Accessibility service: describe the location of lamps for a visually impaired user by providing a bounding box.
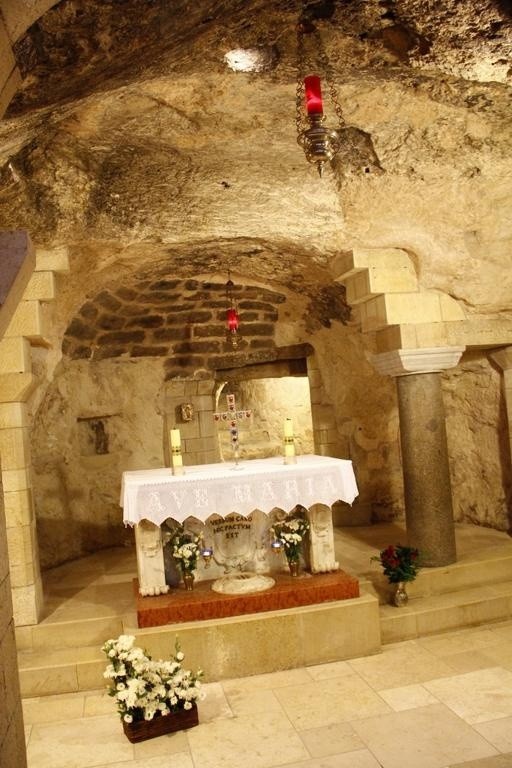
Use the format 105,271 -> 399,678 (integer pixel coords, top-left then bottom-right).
282,416 -> 298,464
220,268 -> 244,353
167,424 -> 185,476
290,0 -> 351,172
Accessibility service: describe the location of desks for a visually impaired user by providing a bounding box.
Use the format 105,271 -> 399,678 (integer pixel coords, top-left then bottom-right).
117,453 -> 359,599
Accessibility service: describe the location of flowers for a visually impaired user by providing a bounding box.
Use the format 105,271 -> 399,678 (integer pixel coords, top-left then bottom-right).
267,513 -> 311,560
369,543 -> 423,583
102,631 -> 208,724
168,530 -> 207,573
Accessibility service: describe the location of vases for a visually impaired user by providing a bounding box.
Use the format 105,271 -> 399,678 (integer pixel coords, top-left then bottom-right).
392,580 -> 408,607
121,701 -> 200,745
182,568 -> 194,590
285,554 -> 301,576
